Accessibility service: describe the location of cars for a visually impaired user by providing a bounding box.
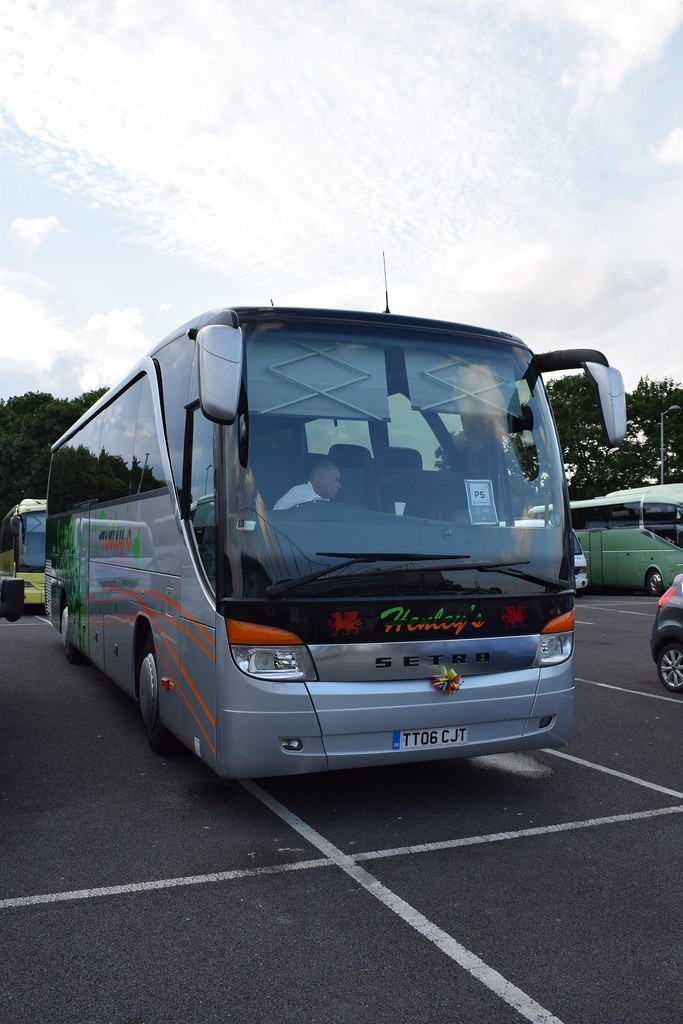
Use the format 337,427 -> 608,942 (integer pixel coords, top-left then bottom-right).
650,573 -> 683,692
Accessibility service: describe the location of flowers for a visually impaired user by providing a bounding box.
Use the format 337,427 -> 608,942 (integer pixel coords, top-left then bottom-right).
431,665 -> 464,696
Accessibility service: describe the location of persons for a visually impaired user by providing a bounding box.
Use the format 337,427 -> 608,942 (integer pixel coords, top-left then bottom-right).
272,463 -> 341,510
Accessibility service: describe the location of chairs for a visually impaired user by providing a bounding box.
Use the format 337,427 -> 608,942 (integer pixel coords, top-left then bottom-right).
328,443 -> 423,472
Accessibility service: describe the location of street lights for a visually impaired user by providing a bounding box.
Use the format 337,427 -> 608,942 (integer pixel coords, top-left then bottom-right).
660,405 -> 680,485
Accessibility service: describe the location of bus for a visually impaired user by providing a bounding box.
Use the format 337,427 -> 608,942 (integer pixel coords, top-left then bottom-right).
527,483 -> 683,597
43,304 -> 627,779
0,498 -> 47,606
